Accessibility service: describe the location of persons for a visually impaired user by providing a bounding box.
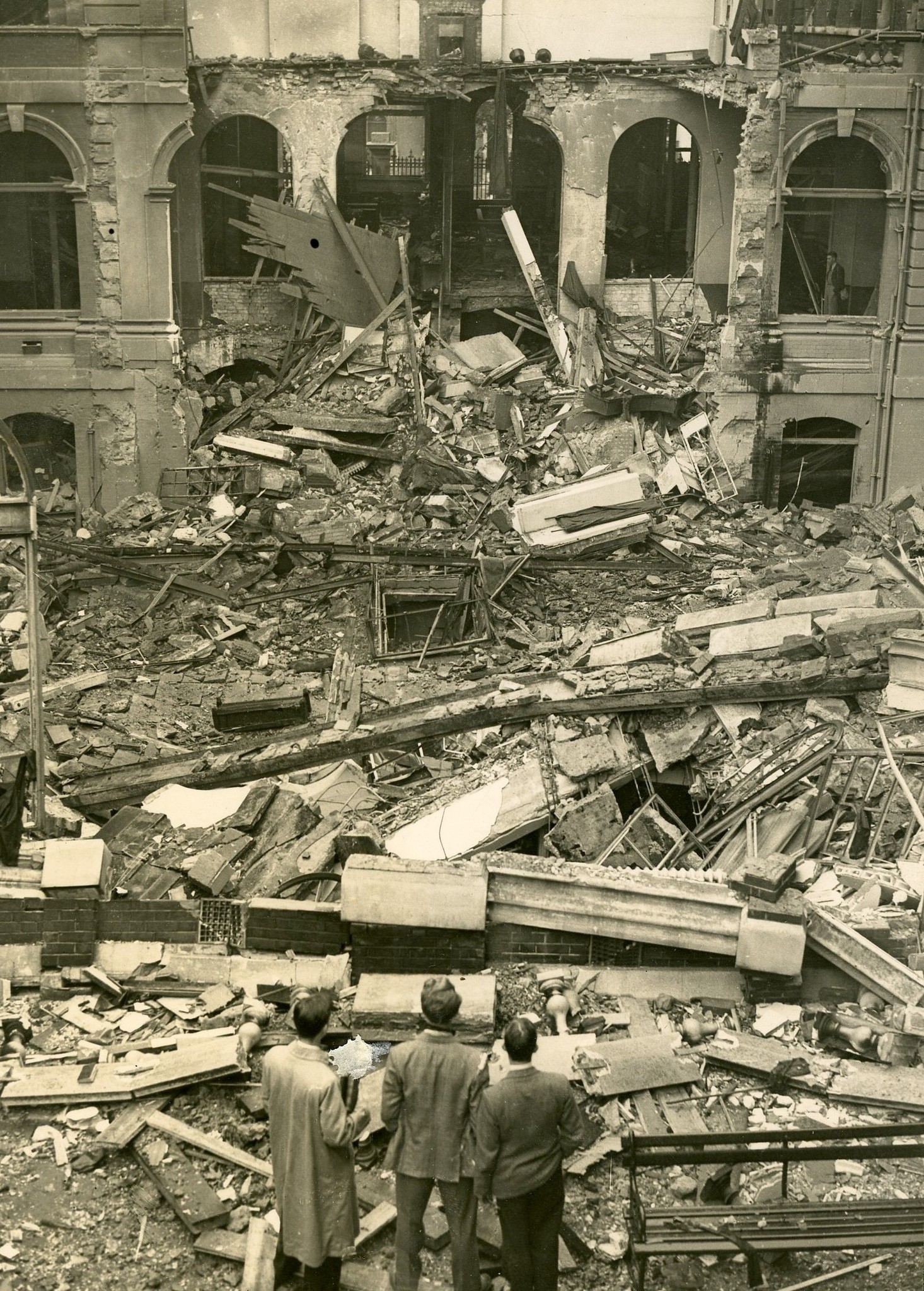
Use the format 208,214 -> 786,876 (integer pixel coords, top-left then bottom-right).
380,978 -> 489,1291
475,1017 -> 584,1291
261,993 -> 372,1291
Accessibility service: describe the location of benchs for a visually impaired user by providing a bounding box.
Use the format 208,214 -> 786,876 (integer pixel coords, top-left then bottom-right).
620,1124 -> 924,1291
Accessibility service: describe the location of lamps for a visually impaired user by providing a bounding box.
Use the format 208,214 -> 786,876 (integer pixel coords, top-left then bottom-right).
22,344 -> 43,353
358,44 -> 375,58
535,49 -> 551,63
508,49 -> 525,63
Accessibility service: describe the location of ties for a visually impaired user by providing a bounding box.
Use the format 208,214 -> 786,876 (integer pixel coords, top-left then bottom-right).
826,265 -> 832,279
